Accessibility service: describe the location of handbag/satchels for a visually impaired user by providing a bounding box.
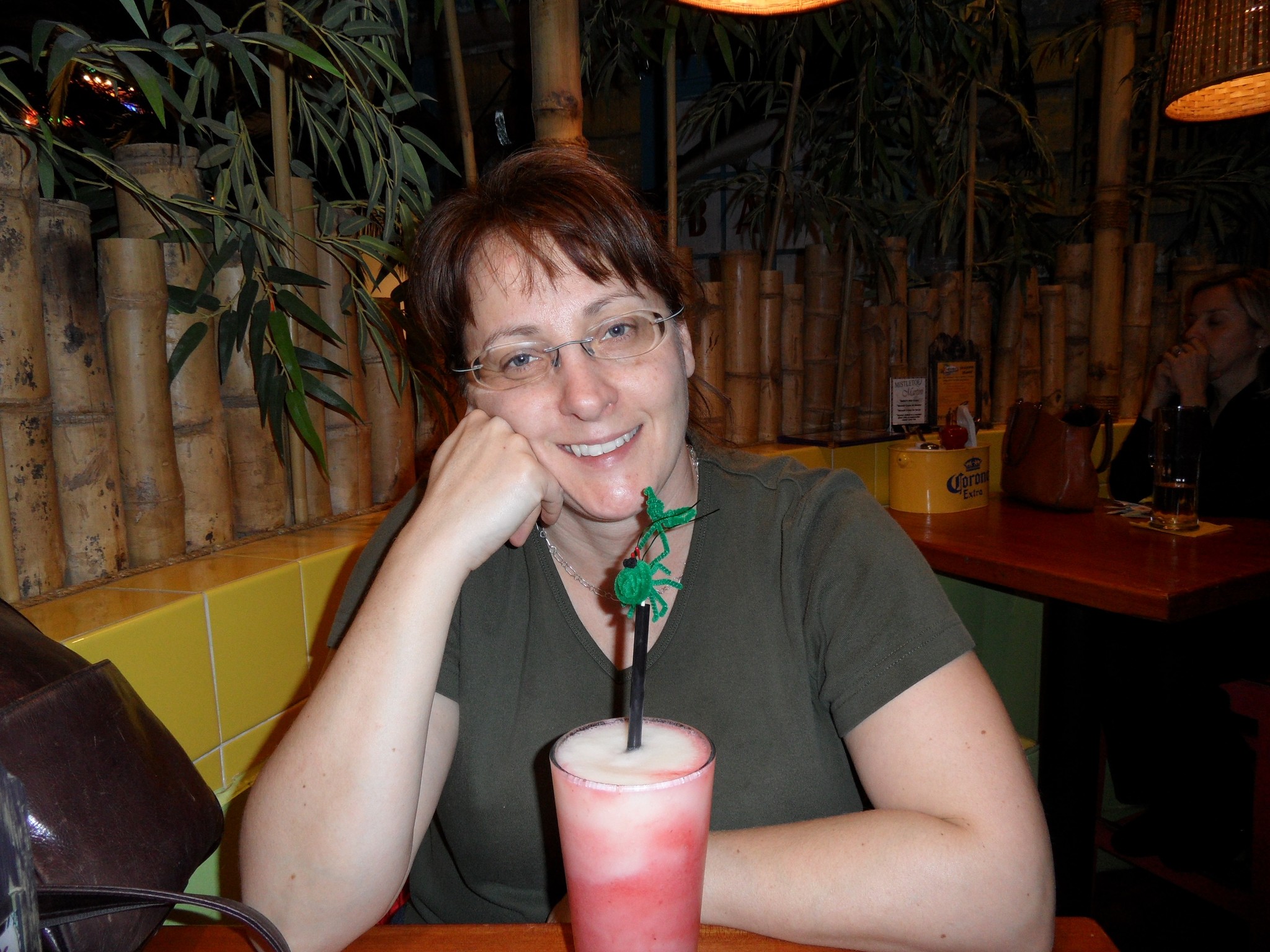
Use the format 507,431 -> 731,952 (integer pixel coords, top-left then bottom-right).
1000,396 -> 1113,510
0,597 -> 292,950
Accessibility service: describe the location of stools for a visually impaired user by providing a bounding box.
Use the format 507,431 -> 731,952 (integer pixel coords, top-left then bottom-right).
1094,668 -> 1270,912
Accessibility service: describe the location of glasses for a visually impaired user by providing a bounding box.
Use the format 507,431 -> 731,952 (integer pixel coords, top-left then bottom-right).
444,303 -> 688,394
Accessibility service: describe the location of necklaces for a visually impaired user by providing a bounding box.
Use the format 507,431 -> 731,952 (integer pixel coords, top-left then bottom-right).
538,444 -> 700,608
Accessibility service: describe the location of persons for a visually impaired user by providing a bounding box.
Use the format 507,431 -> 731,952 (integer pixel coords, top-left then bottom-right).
242,139 -> 1056,952
1110,276 -> 1270,889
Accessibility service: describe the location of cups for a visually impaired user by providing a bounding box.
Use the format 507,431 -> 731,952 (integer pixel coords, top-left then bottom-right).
1150,405 -> 1207,531
549,716 -> 716,952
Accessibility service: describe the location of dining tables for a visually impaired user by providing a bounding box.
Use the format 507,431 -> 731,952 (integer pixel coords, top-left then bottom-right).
137,917 -> 1115,952
863,488 -> 1270,919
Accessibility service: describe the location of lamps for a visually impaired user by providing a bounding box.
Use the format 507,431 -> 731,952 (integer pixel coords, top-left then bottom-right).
1160,0 -> 1270,123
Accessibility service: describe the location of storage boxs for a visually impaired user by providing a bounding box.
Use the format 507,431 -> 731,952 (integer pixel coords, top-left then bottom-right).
888,439 -> 992,514
